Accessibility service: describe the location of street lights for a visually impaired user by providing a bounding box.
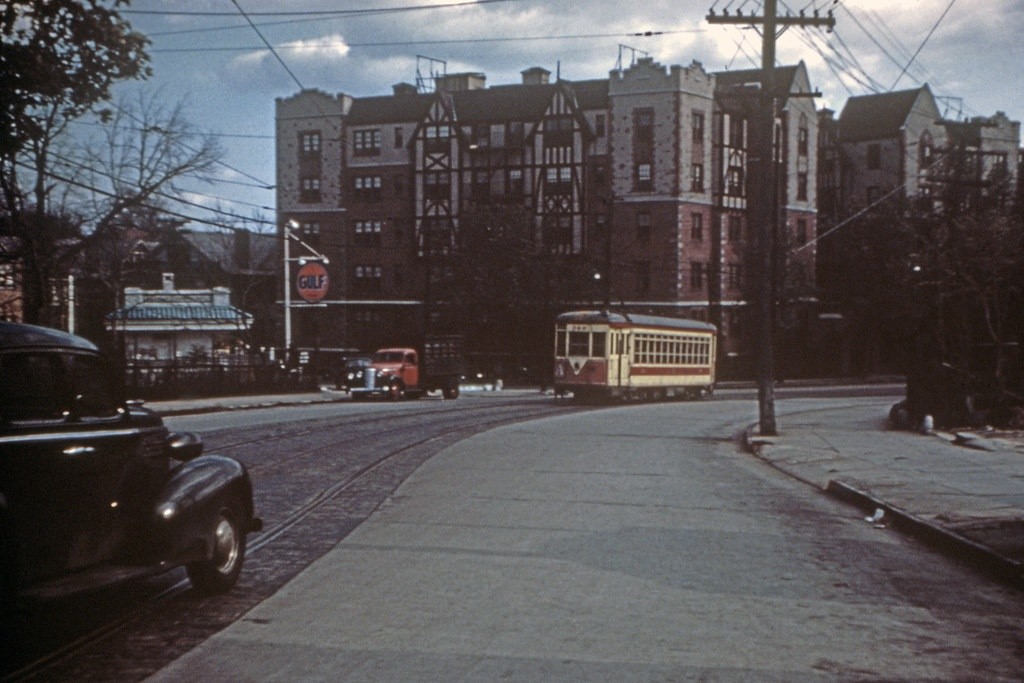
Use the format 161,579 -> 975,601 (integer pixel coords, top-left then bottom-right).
283,219 -> 329,366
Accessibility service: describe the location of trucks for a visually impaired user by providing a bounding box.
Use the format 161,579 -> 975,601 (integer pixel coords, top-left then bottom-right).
364,336 -> 466,399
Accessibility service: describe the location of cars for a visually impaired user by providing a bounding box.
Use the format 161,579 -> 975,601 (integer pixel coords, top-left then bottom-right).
0,322 -> 263,617
337,357 -> 369,390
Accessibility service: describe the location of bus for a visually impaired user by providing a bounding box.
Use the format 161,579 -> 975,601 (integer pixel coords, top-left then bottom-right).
553,262 -> 717,401
